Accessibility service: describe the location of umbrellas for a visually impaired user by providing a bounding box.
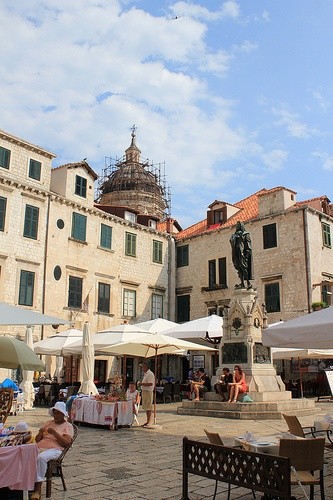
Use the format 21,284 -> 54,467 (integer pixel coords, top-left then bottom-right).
0,302 -> 99,409
99,332 -> 220,425
60,314 -> 223,388
262,305 -> 333,398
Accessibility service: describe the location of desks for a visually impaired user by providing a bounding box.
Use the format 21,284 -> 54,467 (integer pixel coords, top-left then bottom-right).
0,442 -> 40,500
65,395 -> 134,428
155,386 -> 164,397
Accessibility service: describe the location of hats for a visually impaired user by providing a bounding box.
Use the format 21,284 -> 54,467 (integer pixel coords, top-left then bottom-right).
12,422 -> 28,433
48,402 -> 69,418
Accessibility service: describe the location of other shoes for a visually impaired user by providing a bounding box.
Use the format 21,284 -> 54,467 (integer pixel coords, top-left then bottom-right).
141,423 -> 149,428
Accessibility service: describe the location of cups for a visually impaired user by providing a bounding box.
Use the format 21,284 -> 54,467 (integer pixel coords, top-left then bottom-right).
44,427 -> 49,435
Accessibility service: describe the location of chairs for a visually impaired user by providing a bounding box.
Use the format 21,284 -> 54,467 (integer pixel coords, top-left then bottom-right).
44,423 -> 78,496
205,431 -> 257,500
279,437 -> 325,500
158,381 -> 183,403
36,384 -> 57,407
282,414 -> 333,448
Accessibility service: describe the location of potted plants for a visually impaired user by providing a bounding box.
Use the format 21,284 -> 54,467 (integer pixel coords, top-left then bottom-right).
312,302 -> 326,311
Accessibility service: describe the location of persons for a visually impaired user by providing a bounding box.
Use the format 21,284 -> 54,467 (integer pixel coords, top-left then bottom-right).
138,363 -> 155,425
30,402 -> 74,500
230,221 -> 254,289
188,365 -> 247,403
125,384 -> 140,419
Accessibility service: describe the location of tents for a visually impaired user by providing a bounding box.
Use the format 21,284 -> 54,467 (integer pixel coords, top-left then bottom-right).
270,347 -> 333,398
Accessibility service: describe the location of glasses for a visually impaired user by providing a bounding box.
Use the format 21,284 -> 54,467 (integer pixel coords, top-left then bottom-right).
234,369 -> 238,370
